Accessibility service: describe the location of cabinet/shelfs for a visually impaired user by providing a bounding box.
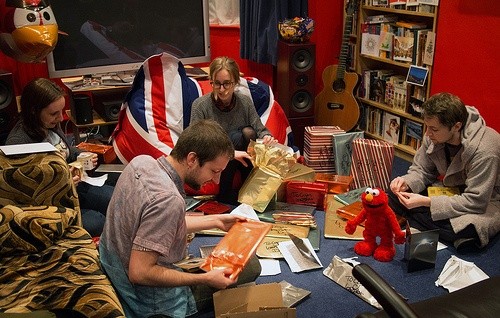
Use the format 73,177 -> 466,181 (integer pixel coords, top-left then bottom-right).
61,64 -> 209,140
353,0 -> 440,163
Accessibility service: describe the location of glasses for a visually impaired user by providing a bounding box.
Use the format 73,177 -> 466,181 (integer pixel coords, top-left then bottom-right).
211,81 -> 237,89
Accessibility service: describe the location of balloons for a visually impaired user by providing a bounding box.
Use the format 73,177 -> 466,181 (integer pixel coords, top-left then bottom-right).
0,0 -> 68,64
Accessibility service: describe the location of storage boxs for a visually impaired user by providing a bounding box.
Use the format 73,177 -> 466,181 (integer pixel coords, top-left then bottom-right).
349,139 -> 394,193
286,181 -> 329,206
302,126 -> 346,174
75,142 -> 116,164
314,173 -> 352,194
238,139 -> 299,213
333,131 -> 364,176
213,282 -> 296,318
278,163 -> 315,201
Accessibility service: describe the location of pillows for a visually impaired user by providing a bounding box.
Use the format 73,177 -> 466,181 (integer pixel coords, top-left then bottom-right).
0,203 -> 79,254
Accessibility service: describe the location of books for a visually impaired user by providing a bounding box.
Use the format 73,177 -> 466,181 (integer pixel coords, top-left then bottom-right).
61,67 -> 209,90
355,0 -> 436,152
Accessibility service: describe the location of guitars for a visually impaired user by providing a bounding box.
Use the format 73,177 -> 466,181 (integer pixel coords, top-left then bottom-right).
313,0 -> 364,133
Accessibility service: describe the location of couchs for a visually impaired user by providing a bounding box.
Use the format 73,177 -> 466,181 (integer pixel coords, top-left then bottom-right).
0,150 -> 125,318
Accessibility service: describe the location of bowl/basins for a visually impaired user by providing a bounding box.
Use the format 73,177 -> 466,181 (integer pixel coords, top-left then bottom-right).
278,18 -> 313,41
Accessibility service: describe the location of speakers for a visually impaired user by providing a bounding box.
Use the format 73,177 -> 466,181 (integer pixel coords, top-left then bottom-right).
72,95 -> 95,126
285,114 -> 316,157
92,92 -> 123,122
0,71 -> 20,148
276,40 -> 317,118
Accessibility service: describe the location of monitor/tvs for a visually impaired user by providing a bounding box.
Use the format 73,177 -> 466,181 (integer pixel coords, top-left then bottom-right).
38,0 -> 212,85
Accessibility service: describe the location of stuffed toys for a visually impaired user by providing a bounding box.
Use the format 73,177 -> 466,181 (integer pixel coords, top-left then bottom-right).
345,188 -> 407,262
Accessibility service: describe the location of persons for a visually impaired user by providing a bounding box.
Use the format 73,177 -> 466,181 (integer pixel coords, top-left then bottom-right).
189,56 -> 278,208
390,94 -> 500,254
98,118 -> 262,318
4,77 -> 114,238
